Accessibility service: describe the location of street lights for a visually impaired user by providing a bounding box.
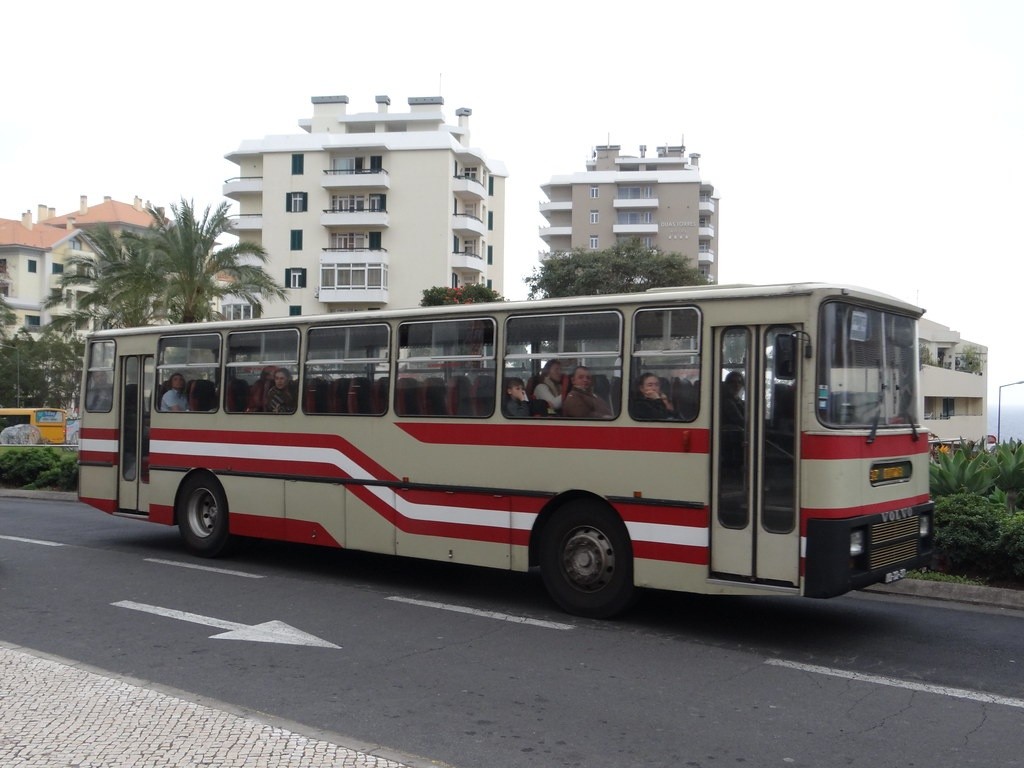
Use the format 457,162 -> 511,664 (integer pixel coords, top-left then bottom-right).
997,380 -> 1024,447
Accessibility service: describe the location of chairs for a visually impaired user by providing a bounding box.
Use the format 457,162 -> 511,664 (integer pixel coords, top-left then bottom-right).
87,365 -> 774,468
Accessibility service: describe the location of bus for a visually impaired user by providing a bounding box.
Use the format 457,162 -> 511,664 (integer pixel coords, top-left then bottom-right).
75,278 -> 936,622
0,407 -> 66,445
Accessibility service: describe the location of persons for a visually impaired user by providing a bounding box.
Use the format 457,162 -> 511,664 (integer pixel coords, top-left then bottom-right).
267,368 -> 297,413
160,373 -> 188,412
86,372 -> 112,410
723,373 -> 745,423
561,366 -> 610,420
533,360 -> 570,418
506,377 -> 548,417
632,373 -> 674,420
